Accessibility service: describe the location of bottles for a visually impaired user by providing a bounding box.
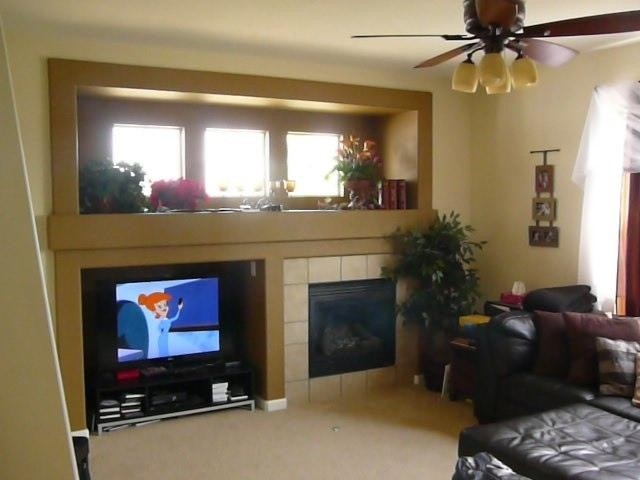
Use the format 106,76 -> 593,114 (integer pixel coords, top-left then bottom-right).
276,176 -> 289,209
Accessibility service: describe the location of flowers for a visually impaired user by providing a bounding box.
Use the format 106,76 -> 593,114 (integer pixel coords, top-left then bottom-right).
325,132 -> 383,179
82,157 -> 147,210
151,179 -> 210,212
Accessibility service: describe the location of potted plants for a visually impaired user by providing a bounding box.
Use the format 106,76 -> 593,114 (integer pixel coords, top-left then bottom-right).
380,211 -> 482,376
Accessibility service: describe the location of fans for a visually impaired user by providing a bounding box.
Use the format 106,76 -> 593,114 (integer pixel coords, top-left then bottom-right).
348,1 -> 640,79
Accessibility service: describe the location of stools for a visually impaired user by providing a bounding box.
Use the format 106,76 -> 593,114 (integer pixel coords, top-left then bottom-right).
457,402 -> 640,480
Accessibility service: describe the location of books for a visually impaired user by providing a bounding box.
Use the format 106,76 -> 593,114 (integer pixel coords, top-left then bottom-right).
99,393 -> 161,431
212,381 -> 249,403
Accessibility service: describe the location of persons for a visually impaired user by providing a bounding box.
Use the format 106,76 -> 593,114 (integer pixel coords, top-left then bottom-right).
138,292 -> 183,357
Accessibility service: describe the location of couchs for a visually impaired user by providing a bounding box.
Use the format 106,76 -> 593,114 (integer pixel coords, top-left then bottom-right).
481,309 -> 640,418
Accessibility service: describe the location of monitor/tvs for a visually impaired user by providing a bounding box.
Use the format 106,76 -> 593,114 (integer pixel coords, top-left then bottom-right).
110,274 -> 225,383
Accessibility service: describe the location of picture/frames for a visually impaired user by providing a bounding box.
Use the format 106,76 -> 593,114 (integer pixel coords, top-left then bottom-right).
527,164 -> 561,245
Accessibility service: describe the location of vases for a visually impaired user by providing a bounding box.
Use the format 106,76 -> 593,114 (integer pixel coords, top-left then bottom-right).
340,179 -> 370,193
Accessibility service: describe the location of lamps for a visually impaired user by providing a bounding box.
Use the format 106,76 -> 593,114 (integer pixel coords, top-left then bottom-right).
455,26 -> 538,94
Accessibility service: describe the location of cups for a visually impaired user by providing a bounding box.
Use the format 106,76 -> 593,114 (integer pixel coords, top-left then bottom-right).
286,179 -> 295,200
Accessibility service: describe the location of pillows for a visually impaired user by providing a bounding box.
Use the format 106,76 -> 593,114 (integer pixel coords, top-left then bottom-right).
534,309 -> 640,404
